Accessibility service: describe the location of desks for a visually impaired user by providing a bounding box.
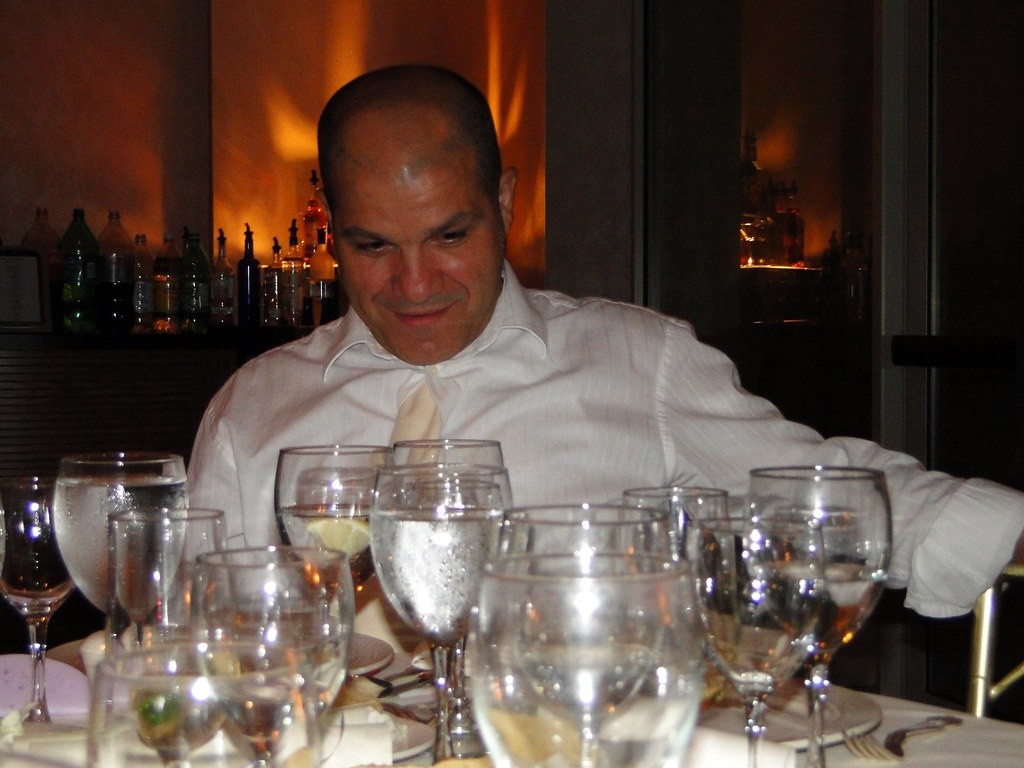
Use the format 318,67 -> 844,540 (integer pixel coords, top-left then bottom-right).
795,688 -> 1024,768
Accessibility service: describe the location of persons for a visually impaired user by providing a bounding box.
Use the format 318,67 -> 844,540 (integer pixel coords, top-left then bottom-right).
185,62 -> 1024,653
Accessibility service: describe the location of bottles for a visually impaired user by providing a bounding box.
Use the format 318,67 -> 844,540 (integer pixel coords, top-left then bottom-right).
238,223 -> 260,326
21,206 -> 62,329
742,126 -> 805,265
59,209 -> 103,329
181,229 -> 211,328
264,219 -> 314,328
213,228 -> 237,327
98,209 -> 136,329
133,231 -> 154,331
155,233 -> 181,332
302,170 -> 349,327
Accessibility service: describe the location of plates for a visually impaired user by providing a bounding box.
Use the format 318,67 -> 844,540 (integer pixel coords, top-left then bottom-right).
80,632 -> 394,673
377,708 -> 437,762
694,679 -> 882,750
1,654 -> 89,726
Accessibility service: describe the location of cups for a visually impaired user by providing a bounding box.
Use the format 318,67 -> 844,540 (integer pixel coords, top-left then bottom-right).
86,507 -> 355,768
464,503 -> 704,768
620,486 -> 730,704
740,212 -> 777,260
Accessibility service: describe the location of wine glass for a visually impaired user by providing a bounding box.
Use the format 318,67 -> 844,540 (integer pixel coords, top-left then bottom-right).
370,439 -> 514,768
0,477 -> 77,740
275,441 -> 394,641
52,451 -> 188,649
749,465 -> 892,768
683,518 -> 826,768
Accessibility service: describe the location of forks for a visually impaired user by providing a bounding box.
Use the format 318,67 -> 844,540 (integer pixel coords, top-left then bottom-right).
839,715 -> 963,759
